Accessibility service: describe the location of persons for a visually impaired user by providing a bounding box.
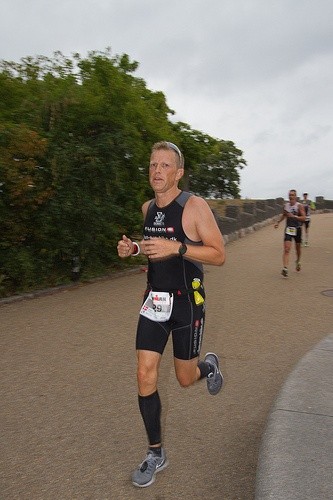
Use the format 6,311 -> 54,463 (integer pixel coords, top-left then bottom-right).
300,193 -> 315,247
273,190 -> 305,277
116,141 -> 225,487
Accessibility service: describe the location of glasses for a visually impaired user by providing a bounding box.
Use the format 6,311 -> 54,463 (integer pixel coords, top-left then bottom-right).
165,142 -> 182,161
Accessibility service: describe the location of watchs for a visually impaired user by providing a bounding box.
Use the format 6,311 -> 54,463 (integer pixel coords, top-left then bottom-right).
179,241 -> 187,257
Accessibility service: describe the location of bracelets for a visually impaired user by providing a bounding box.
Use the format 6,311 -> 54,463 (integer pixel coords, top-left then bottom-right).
130,242 -> 140,256
294,215 -> 297,218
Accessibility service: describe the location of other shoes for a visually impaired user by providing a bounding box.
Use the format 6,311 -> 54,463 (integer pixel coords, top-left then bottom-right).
296,265 -> 302,271
280,269 -> 289,279
304,241 -> 308,246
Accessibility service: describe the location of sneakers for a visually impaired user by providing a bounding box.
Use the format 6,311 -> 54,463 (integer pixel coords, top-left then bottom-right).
132,448 -> 168,488
203,352 -> 223,396
